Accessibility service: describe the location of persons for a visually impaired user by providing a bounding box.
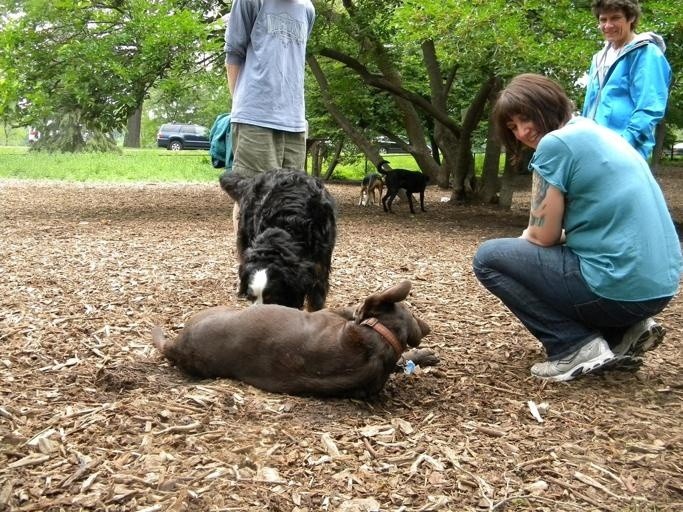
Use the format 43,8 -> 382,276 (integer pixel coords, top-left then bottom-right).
470,73 -> 682,385
580,0 -> 672,162
223,0 -> 316,237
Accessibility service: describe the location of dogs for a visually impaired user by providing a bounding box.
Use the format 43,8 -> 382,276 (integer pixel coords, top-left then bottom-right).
151,278 -> 440,399
377,160 -> 430,214
358,173 -> 386,208
220,167 -> 336,312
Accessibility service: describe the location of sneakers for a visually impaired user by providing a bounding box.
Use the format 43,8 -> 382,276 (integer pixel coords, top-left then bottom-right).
531,334 -> 616,384
606,317 -> 665,372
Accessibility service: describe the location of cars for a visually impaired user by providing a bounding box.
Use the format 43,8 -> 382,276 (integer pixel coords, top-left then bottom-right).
370,133 -> 434,156
27,118 -> 90,148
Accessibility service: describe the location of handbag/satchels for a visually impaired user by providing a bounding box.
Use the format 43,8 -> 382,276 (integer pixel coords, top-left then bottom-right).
209,114 -> 235,169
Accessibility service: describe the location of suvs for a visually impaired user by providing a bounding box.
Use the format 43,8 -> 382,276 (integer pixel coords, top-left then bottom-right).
156,119 -> 214,150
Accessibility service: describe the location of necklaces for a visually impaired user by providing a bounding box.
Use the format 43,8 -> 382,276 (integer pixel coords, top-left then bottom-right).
602,40 -> 629,83
